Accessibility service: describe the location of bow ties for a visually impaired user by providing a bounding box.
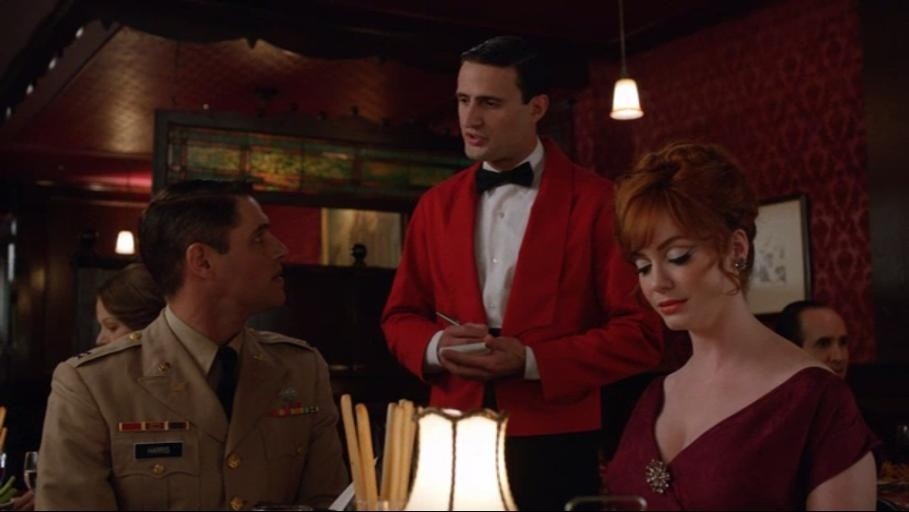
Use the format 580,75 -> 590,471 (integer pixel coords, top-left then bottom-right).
476,160 -> 534,192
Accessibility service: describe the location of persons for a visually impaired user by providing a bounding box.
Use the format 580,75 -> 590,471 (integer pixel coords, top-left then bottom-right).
601,138 -> 880,512
36,176 -> 356,511
379,37 -> 673,509
94,263 -> 166,349
776,300 -> 849,386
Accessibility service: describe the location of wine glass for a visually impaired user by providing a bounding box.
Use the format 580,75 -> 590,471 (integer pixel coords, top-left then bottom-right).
22,450 -> 39,491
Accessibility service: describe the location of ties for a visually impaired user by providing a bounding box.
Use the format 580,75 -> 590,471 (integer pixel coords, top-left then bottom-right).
206,345 -> 239,421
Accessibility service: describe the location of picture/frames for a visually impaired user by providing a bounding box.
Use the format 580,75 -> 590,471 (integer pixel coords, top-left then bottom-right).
742,192 -> 812,319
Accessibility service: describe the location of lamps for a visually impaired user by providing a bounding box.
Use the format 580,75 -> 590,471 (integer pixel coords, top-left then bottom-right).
606,0 -> 646,122
113,159 -> 136,255
402,407 -> 520,512
